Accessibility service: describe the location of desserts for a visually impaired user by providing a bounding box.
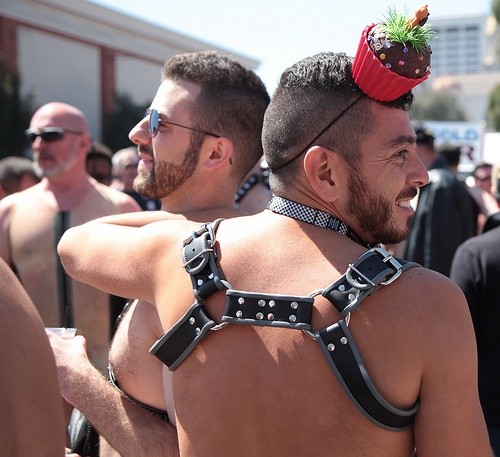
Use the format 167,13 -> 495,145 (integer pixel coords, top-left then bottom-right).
353,3 -> 437,103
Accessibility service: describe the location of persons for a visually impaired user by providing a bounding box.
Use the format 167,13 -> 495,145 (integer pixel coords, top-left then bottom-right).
0,102 -> 143,446
0,258 -> 67,457
56,52 -> 493,457
451,164 -> 500,456
46,51 -> 271,457
0,126 -> 500,297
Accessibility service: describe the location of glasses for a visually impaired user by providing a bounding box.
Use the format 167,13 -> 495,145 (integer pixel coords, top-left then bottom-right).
144,108 -> 232,165
28,127 -> 81,142
